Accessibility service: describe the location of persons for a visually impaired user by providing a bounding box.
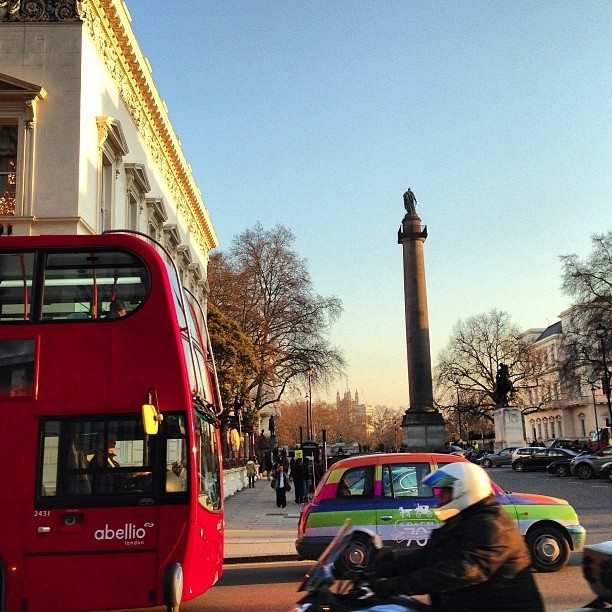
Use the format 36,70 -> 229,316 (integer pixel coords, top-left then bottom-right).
89,431 -> 128,493
302,458 -> 311,502
292,458 -> 304,503
259,464 -> 263,473
283,460 -> 289,474
105,300 -> 127,320
350,461 -> 546,612
271,466 -> 291,508
290,458 -> 295,468
336,477 -> 351,499
310,459 -> 324,492
337,447 -> 345,455
264,458 -> 273,481
247,457 -> 257,488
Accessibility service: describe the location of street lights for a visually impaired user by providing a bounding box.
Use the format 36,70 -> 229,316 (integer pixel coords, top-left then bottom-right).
590,385 -> 600,449
455,381 -> 461,438
306,365 -> 313,441
305,393 -> 310,440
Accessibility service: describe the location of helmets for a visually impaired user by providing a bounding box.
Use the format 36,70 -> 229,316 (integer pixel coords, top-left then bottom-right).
419,462 -> 495,523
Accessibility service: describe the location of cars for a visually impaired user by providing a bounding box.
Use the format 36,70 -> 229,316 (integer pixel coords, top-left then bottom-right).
477,446 -> 521,467
569,445 -> 611,478
546,450 -> 597,477
511,446 -> 546,463
432,445 -> 462,452
512,448 -> 576,471
295,453 -> 586,572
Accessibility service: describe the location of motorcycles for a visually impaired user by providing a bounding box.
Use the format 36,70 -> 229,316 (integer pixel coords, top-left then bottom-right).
290,518 -> 611,611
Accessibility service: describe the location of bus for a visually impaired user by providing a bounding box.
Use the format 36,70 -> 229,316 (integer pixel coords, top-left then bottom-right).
0,229 -> 244,611
588,427 -> 611,450
